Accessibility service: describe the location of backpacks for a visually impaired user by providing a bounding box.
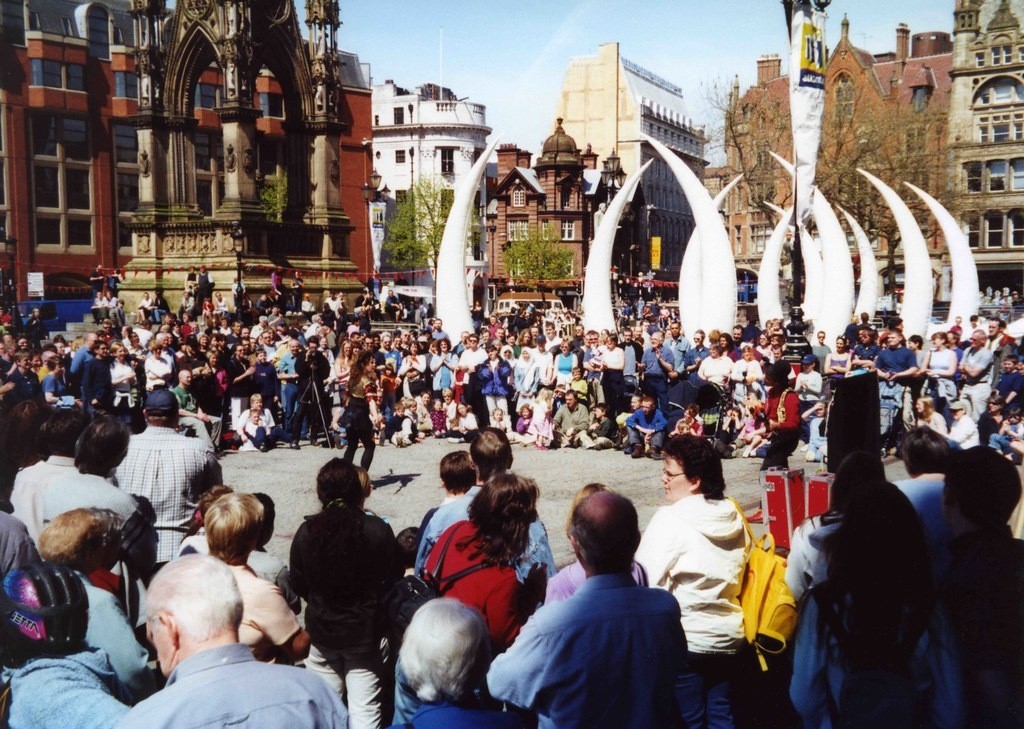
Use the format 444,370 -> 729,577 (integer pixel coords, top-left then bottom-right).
933,526 -> 1024,661
813,486 -> 939,678
386,526 -> 508,656
722,494 -> 803,672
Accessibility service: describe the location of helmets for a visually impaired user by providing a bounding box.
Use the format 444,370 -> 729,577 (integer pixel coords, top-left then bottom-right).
3,563 -> 92,655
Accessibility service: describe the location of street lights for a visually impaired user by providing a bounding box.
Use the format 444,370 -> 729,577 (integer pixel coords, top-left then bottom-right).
607,148 -> 619,198
2,236 -> 18,337
232,230 -> 246,307
362,168 -> 390,320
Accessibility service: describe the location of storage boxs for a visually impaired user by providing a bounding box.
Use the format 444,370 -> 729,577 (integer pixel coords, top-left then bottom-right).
804,471 -> 835,518
758,465 -> 806,552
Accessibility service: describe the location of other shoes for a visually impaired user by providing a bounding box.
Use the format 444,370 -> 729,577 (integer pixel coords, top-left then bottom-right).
290,440 -> 300,450
397,435 -> 405,447
334,432 -> 343,448
259,442 -> 267,452
632,444 -> 661,457
310,439 -> 319,446
742,448 -> 751,459
751,449 -> 757,455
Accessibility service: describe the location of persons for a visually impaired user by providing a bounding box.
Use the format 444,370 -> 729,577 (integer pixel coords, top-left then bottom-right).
243,144 -> 256,173
225,144 -> 236,172
140,150 -> 151,177
0,266 -> 1024,729
329,157 -> 339,188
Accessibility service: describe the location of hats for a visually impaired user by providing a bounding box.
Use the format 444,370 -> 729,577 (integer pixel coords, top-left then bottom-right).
764,360 -> 792,385
801,354 -> 816,364
145,390 -> 177,409
949,401 -> 967,410
537,335 -> 545,342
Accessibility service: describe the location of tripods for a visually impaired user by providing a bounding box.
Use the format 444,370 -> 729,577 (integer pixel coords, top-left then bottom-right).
282,361 -> 333,450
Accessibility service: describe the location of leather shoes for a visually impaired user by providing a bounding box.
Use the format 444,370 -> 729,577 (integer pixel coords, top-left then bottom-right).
745,511 -> 763,524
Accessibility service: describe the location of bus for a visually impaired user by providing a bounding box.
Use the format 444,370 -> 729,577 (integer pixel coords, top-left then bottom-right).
498,293 -> 566,319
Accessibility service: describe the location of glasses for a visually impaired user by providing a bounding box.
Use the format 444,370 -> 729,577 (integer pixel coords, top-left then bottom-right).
970,337 -> 979,341
659,467 -> 686,482
